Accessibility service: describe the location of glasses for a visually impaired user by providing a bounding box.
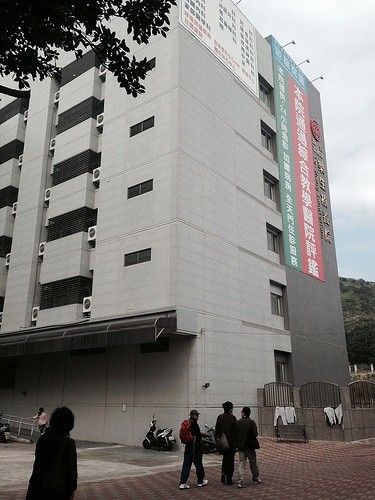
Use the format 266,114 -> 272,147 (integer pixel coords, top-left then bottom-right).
195,414 -> 201,417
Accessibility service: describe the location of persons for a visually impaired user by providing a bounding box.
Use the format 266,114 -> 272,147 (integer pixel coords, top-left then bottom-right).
29,408 -> 48,436
234,407 -> 262,488
179,410 -> 208,489
215,401 -> 237,485
26,407 -> 77,500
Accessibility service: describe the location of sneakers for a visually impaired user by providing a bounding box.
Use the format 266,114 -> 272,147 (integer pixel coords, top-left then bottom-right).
179,483 -> 191,489
252,477 -> 262,484
236,481 -> 246,488
197,479 -> 209,487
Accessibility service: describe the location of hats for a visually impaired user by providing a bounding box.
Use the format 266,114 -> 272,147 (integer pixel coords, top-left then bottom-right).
187,409 -> 201,416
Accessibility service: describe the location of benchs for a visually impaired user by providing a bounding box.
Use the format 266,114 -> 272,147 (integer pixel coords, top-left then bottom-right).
275,425 -> 309,444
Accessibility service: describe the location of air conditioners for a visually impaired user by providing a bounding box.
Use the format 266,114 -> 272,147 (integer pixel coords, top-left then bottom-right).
0,312 -> 3,326
44,188 -> 53,201
32,306 -> 39,322
92,167 -> 102,183
54,90 -> 60,103
38,241 -> 47,255
99,63 -> 108,76
17,154 -> 24,167
6,253 -> 11,265
23,110 -> 28,122
96,113 -> 104,128
12,202 -> 17,214
87,226 -> 97,242
49,138 -> 55,150
83,296 -> 93,313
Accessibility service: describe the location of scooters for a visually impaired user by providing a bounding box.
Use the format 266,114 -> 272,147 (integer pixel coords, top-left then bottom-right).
0,414 -> 11,443
201,423 -> 218,454
142,413 -> 176,452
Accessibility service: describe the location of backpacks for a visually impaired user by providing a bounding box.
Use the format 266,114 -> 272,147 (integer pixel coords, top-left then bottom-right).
179,419 -> 195,444
214,427 -> 231,452
